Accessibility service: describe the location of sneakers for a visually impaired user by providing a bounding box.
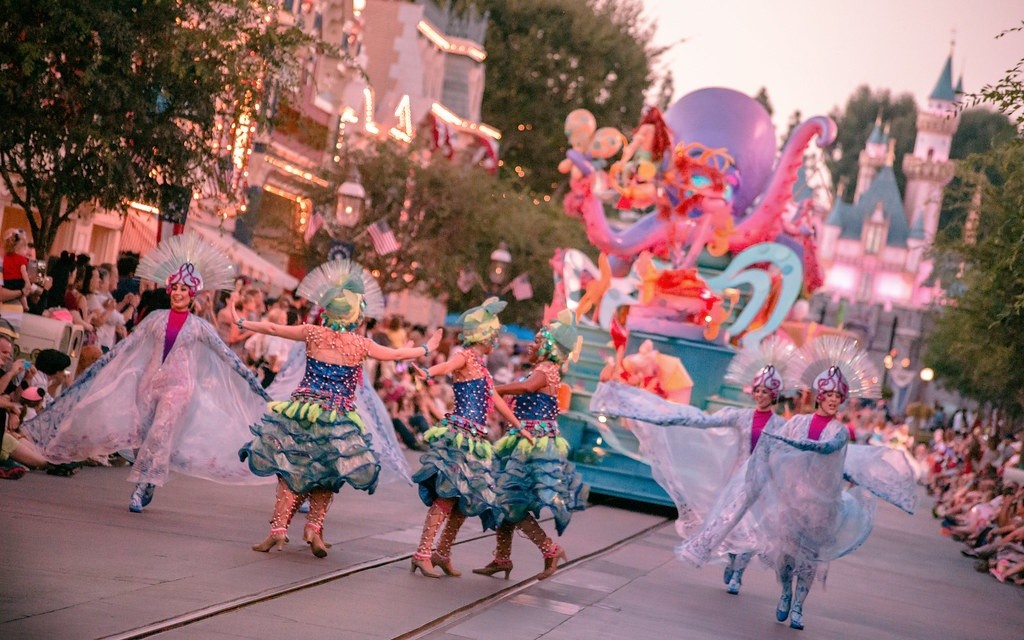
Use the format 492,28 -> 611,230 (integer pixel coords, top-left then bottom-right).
0,457 -> 25,480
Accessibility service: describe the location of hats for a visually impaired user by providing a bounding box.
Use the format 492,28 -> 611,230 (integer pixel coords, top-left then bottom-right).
132,231 -> 236,297
536,309 -> 577,367
723,336 -> 809,404
295,260 -> 385,334
20,380 -> 46,401
456,296 -> 508,349
784,335 -> 883,408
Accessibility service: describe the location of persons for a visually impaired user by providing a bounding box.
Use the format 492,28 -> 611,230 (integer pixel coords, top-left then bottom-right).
411,304 -> 535,581
78,268 -> 256,513
473,322 -> 580,583
774,385 -> 1024,588
0,225 -> 541,480
225,284 -> 443,560
661,363 -> 850,630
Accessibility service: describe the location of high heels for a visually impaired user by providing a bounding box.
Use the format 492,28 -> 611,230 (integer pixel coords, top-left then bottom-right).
252,528 -> 286,552
430,553 -> 462,577
409,557 -> 441,578
538,547 -> 568,581
304,527 -> 328,558
472,562 -> 512,580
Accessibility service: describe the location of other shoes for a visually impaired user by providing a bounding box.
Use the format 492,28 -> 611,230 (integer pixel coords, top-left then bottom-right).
728,578 -> 741,594
776,595 -> 792,621
45,461 -> 82,477
724,567 -> 735,583
141,483 -> 155,507
791,610 -> 804,630
928,479 -> 1009,584
129,491 -> 144,513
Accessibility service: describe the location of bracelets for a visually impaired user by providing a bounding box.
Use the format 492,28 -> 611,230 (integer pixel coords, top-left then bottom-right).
421,344 -> 429,356
421,367 -> 431,381
237,317 -> 245,329
514,424 -> 524,432
